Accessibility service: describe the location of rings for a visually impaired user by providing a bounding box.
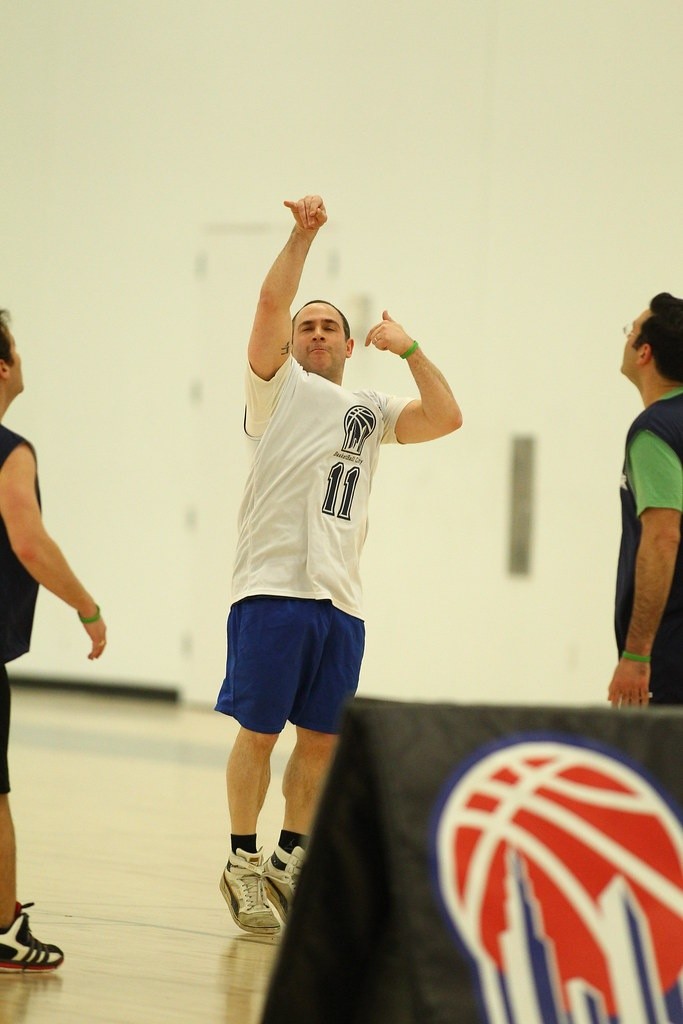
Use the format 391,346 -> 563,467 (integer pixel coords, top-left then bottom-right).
97,639 -> 106,647
374,337 -> 378,342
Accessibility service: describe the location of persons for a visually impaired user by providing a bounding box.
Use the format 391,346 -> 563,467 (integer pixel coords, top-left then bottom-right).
605,289 -> 679,711
214,197 -> 465,935
0,313 -> 111,981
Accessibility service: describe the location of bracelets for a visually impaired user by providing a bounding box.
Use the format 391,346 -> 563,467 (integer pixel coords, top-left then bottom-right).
619,651 -> 652,666
77,604 -> 101,625
401,340 -> 418,359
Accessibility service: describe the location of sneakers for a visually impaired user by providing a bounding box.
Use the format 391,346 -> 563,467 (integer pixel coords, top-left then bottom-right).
218,848 -> 280,933
264,848 -> 308,926
0,901 -> 65,972
